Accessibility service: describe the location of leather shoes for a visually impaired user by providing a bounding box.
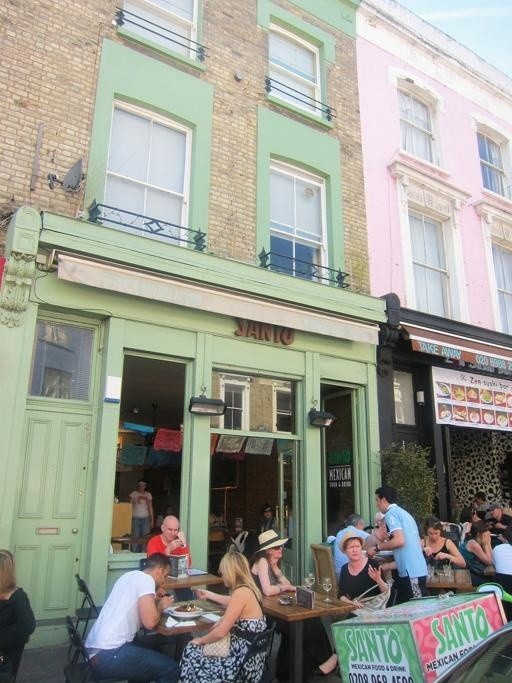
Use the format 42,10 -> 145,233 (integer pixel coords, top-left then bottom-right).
312,655 -> 339,677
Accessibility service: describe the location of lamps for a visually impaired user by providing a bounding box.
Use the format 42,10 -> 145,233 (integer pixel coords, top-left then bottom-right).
308,400 -> 338,427
415,384 -> 427,409
188,385 -> 228,417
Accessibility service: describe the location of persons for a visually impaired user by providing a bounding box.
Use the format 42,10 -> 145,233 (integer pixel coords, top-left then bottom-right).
0,550 -> 36,683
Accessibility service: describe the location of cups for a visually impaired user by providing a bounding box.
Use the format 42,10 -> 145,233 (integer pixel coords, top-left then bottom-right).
443,565 -> 452,577
427,565 -> 434,577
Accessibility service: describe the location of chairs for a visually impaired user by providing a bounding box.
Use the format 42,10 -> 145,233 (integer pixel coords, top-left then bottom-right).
74,573 -> 103,641
385,588 -> 397,607
111,537 -> 148,553
63,616 -> 95,683
9,637 -> 26,683
209,524 -> 228,575
364,526 -> 379,533
233,621 -> 276,683
140,559 -> 147,571
310,544 -> 339,599
436,523 -> 462,547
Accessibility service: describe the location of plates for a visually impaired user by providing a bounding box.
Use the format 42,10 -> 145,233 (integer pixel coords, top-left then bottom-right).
169,603 -> 203,619
361,550 -> 393,556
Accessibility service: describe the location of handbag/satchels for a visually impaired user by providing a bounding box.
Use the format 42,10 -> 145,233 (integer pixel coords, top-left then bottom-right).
203,628 -> 232,658
351,582 -> 392,617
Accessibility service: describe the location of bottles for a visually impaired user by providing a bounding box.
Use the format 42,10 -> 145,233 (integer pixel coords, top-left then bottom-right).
278,593 -> 291,607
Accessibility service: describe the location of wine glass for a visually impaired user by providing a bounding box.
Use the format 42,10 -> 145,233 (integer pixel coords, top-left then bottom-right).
322,578 -> 332,602
304,572 -> 315,590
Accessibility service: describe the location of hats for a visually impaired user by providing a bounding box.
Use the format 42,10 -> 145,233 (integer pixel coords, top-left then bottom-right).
338,530 -> 365,553
486,503 -> 502,512
254,529 -> 290,553
